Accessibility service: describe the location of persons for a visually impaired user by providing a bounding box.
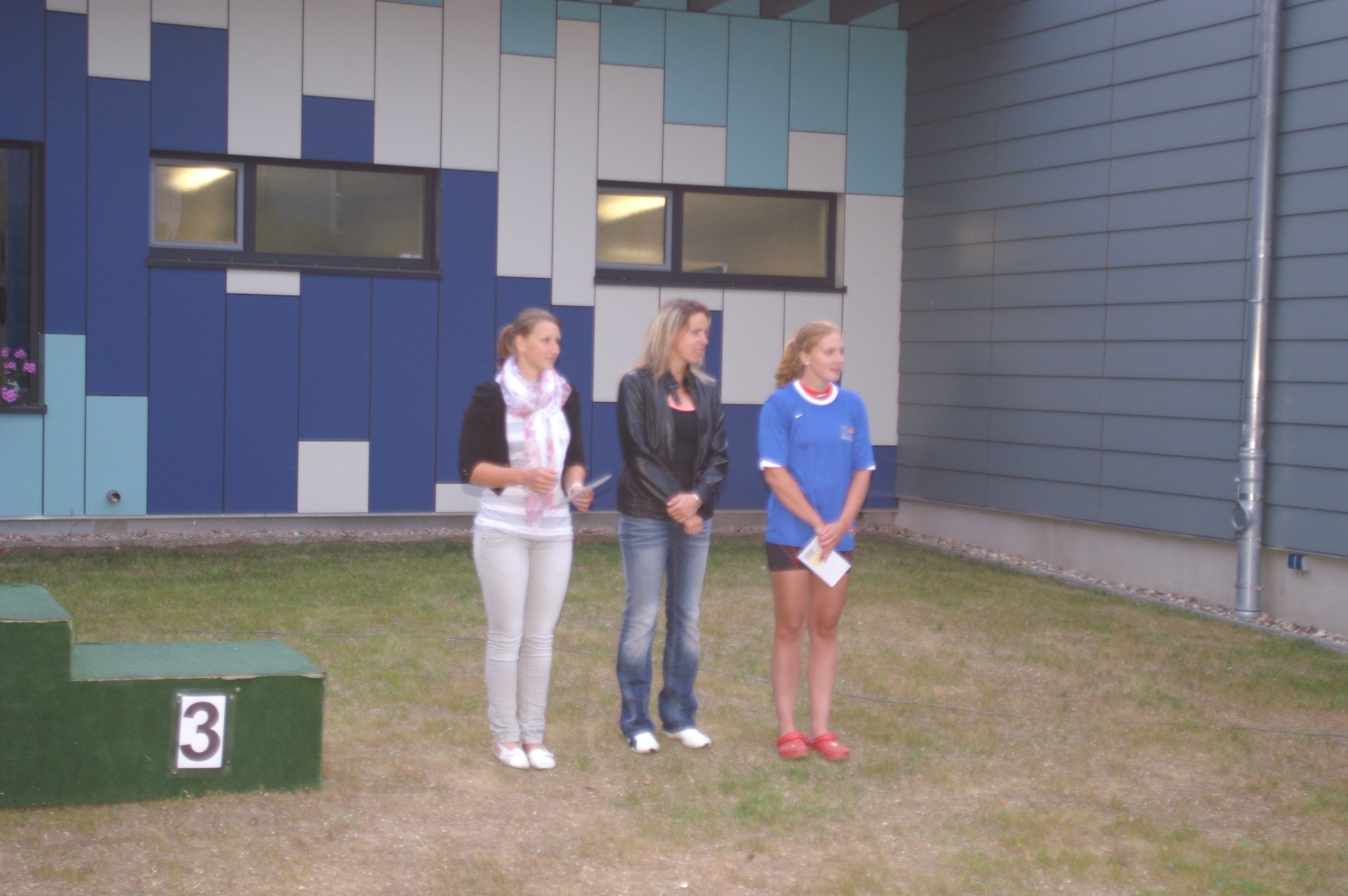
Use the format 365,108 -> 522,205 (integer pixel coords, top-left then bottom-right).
616,298 -> 728,753
458,307 -> 594,770
758,322 -> 876,760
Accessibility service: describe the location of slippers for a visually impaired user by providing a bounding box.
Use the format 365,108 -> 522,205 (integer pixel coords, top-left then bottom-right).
809,732 -> 851,762
777,731 -> 809,758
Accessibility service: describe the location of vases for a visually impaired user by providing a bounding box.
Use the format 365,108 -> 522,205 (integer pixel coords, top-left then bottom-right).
0,383 -> 17,406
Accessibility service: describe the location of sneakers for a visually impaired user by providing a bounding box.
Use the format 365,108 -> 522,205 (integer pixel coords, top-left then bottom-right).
663,729 -> 711,749
627,732 -> 659,755
491,740 -> 556,770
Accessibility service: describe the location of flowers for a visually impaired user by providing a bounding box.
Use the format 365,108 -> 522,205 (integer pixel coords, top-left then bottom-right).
0,346 -> 37,403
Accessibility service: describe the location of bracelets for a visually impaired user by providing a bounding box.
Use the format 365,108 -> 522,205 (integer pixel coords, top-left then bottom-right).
692,493 -> 702,511
566,484 -> 582,497
